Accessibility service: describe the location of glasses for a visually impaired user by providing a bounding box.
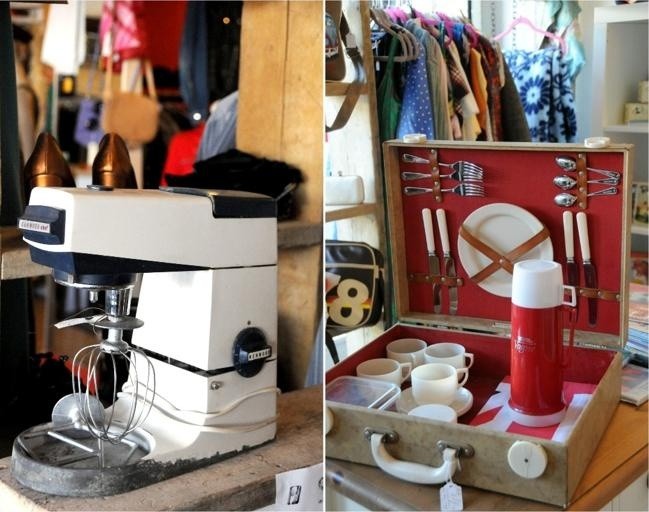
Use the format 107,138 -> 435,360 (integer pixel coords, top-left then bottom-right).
85,100 -> 101,130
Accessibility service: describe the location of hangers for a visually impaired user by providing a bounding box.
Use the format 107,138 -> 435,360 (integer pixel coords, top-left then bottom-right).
364,0 -> 569,64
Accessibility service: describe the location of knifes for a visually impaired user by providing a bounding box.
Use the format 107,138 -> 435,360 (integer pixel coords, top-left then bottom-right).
575,211 -> 598,326
419,207 -> 443,314
561,210 -> 580,313
436,208 -> 460,316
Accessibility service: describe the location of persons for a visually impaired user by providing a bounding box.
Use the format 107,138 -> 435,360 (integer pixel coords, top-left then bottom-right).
11,24 -> 39,172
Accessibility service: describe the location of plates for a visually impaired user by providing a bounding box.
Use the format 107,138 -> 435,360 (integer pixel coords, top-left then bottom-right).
392,383 -> 475,421
454,200 -> 557,299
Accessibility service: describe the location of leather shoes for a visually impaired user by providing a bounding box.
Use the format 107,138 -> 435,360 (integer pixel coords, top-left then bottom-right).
24,132 -> 76,201
92,133 -> 138,187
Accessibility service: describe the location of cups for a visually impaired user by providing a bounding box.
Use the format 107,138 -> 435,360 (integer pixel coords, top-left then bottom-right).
354,336 -> 475,425
505,259 -> 577,430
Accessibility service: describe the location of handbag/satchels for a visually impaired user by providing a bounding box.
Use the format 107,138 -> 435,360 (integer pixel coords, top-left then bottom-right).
74,97 -> 105,146
326,0 -> 366,132
326,239 -> 384,329
103,89 -> 163,147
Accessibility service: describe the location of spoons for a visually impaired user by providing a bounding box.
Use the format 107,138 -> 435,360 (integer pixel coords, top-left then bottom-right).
552,155 -> 620,208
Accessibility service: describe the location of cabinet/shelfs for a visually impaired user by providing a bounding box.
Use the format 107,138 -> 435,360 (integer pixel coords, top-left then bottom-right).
322,2 -> 393,381
586,4 -> 649,361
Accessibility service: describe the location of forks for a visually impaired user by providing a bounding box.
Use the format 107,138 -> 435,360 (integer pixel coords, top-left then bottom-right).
401,153 -> 486,199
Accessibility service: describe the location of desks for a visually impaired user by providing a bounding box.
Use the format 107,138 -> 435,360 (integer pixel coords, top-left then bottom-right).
326,405 -> 647,512
